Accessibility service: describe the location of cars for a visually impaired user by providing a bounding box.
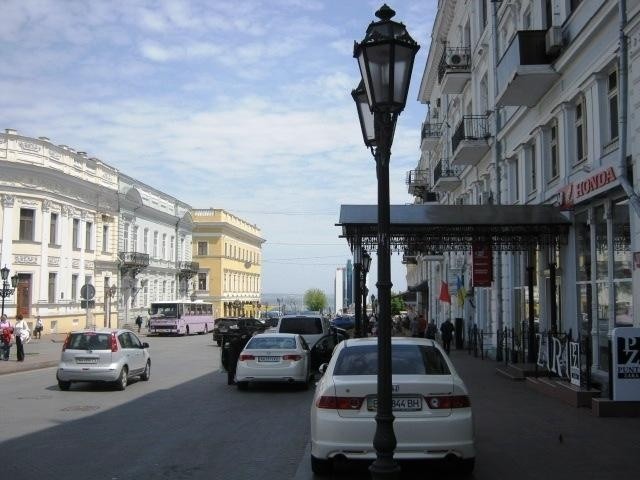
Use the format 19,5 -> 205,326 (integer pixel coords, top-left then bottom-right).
259,317 -> 279,327
56,329 -> 152,391
219,331 -> 341,391
310,338 -> 476,479
332,316 -> 356,330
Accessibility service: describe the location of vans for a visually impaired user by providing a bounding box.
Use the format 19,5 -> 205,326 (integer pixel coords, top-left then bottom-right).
276,314 -> 331,350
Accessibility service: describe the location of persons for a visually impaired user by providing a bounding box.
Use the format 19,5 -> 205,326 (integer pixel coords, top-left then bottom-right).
0,314 -> 12,361
227,333 -> 248,385
135,316 -> 143,333
15,314 -> 29,362
370,314 -> 455,353
35,316 -> 43,339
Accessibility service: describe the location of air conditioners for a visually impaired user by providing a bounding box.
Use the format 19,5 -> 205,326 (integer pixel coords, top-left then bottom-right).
446,54 -> 470,65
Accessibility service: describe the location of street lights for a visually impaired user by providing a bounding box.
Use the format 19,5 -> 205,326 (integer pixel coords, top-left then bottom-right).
360,247 -> 372,336
350,3 -> 421,479
0,263 -> 19,316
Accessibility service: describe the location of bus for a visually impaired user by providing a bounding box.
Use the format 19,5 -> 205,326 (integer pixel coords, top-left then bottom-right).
148,301 -> 215,336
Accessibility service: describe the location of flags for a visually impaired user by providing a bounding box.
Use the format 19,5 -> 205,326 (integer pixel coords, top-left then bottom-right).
440,283 -> 450,305
456,277 -> 466,307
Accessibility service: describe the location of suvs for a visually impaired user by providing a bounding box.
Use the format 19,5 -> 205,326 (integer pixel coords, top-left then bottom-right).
213,317 -> 266,347
